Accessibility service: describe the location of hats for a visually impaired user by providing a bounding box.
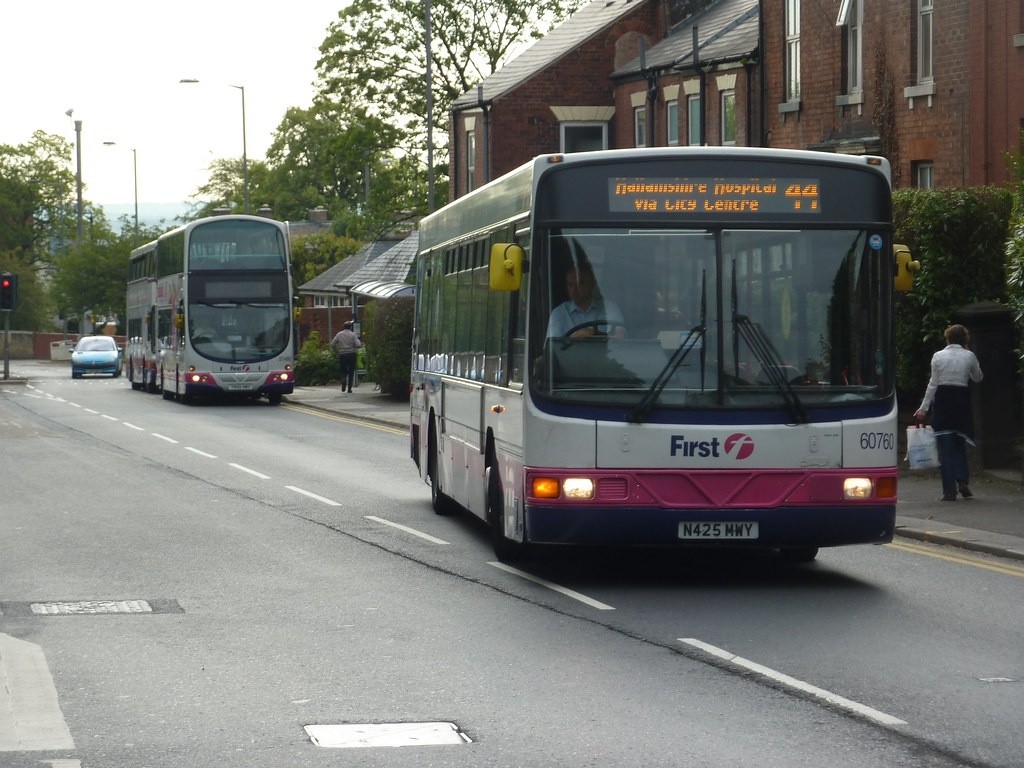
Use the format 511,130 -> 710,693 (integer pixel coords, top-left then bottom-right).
344,321 -> 352,328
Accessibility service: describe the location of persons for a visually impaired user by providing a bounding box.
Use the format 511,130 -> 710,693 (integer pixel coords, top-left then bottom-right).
332,320 -> 361,393
541,262 -> 626,348
193,316 -> 218,339
912,325 -> 982,502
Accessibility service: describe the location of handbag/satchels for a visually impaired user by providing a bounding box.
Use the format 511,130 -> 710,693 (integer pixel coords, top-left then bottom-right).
906,418 -> 941,469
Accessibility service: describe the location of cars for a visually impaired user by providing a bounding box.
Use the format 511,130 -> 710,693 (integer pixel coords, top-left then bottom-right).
69,336 -> 123,377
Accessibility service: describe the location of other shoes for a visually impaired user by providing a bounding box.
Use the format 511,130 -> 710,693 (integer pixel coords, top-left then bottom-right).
941,494 -> 956,501
348,391 -> 352,393
958,484 -> 973,497
342,385 -> 345,392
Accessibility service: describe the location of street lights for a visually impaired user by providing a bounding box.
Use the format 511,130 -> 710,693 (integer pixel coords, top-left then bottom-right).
66,109 -> 84,337
179,79 -> 249,217
103,141 -> 138,244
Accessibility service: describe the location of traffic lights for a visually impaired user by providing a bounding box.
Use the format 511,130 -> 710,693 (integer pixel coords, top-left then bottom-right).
0,274 -> 14,312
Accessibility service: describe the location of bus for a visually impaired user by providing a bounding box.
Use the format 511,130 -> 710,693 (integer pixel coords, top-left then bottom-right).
124,212 -> 302,404
408,145 -> 921,569
